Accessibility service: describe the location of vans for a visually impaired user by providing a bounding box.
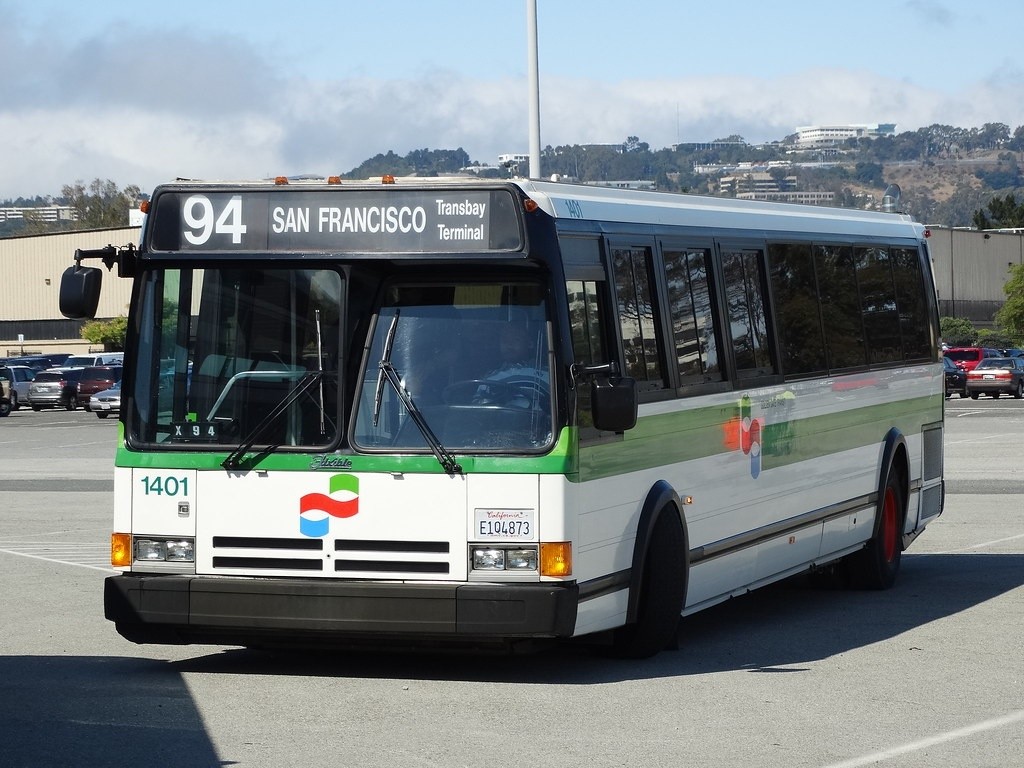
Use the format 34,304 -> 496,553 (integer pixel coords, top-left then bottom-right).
64,352 -> 124,367
27,368 -> 86,412
76,365 -> 123,410
944,348 -> 1002,397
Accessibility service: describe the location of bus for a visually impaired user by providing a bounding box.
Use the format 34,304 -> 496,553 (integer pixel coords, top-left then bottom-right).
57,174 -> 945,654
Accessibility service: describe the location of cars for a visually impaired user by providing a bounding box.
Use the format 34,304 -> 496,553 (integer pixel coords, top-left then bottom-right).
0,354 -> 73,417
1000,350 -> 1024,357
966,357 -> 1024,399
943,356 -> 967,398
90,371 -> 190,419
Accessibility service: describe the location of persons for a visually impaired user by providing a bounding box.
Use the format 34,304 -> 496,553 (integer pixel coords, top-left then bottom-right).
471,319 -> 551,413
